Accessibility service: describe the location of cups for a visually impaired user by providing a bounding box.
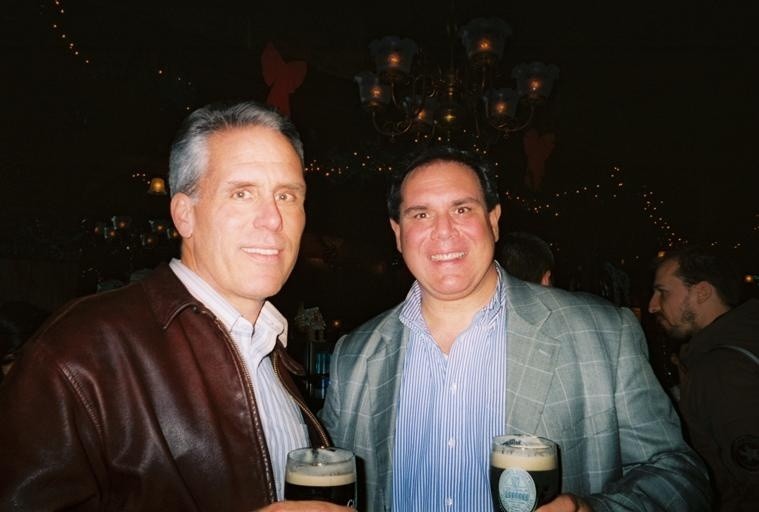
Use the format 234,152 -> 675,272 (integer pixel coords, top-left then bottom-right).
283,447 -> 358,512
488,433 -> 563,512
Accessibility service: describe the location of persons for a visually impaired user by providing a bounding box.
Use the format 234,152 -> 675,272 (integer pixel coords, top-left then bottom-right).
496,231 -> 559,288
316,141 -> 714,511
1,106 -> 356,511
645,243 -> 759,511
